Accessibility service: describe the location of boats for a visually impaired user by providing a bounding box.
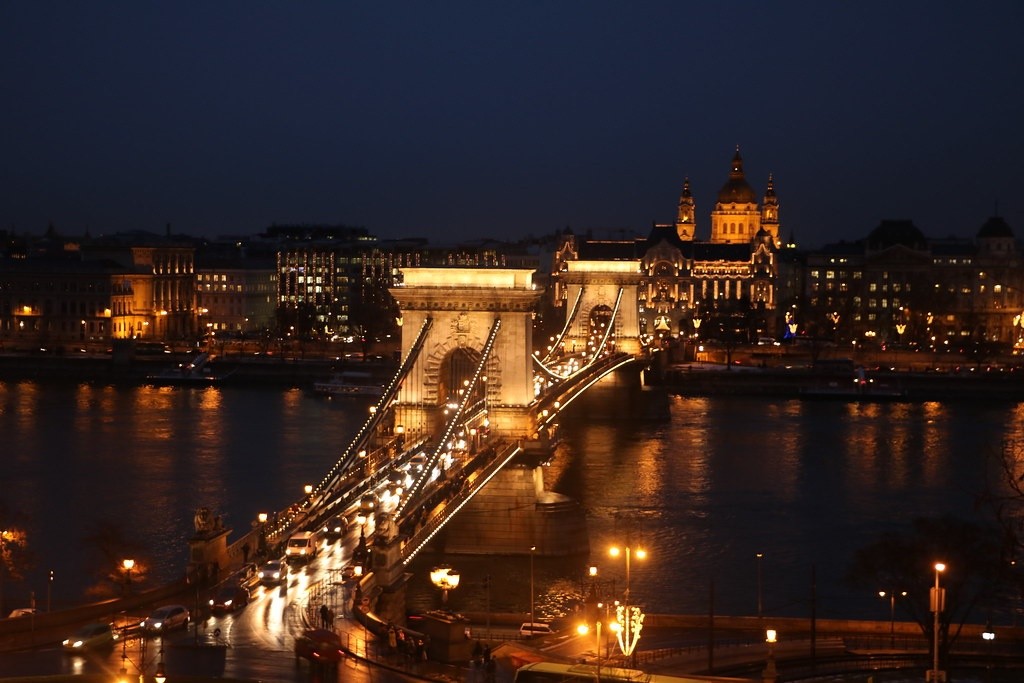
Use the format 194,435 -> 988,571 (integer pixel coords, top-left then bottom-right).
682,351 -> 1024,396
147,352 -> 329,387
314,360 -> 388,395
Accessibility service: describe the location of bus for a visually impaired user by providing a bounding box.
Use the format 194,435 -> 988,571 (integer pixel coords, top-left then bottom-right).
513,662 -> 711,683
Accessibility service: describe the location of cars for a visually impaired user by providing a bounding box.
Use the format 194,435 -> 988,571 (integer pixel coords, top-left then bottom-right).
407,456 -> 428,476
520,622 -> 555,636
260,560 -> 288,585
295,629 -> 344,668
325,515 -> 349,537
209,587 -> 251,610
386,471 -> 407,489
285,531 -> 318,565
140,604 -> 191,635
61,622 -> 114,655
361,491 -> 380,512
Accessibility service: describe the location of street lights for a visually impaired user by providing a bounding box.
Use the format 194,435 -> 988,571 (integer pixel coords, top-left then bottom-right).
879,589 -> 908,649
530,544 -> 536,638
589,566 -> 598,603
605,542 -> 649,660
757,553 -> 763,614
934,562 -> 945,682
